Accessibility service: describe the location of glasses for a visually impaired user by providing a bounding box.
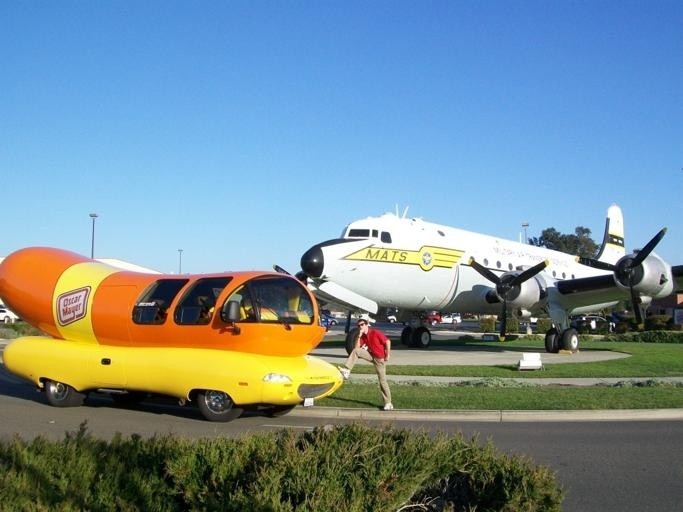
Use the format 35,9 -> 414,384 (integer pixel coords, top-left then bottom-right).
358,323 -> 365,328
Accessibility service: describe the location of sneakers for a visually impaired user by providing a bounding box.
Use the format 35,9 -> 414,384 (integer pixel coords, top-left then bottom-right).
338,366 -> 350,379
384,403 -> 394,410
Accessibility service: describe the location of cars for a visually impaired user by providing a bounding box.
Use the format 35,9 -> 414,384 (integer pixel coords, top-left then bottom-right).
0,304 -> 6,308
0,308 -> 18,324
320,312 -> 340,327
438,314 -> 461,325
569,315 -> 616,335
426,311 -> 442,327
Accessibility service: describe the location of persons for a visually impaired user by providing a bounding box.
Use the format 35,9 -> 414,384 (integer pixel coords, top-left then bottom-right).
338,319 -> 394,411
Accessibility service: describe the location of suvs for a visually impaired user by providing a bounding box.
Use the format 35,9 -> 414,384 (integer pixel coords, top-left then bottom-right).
581,313 -> 621,327
387,310 -> 399,323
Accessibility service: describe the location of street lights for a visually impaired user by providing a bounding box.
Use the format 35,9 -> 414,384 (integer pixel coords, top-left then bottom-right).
177,249 -> 183,274
89,214 -> 99,260
522,220 -> 530,243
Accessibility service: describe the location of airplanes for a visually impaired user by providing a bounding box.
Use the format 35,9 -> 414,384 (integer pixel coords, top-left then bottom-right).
300,200 -> 674,357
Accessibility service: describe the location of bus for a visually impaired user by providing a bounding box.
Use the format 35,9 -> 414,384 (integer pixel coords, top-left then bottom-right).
1,246 -> 347,427
